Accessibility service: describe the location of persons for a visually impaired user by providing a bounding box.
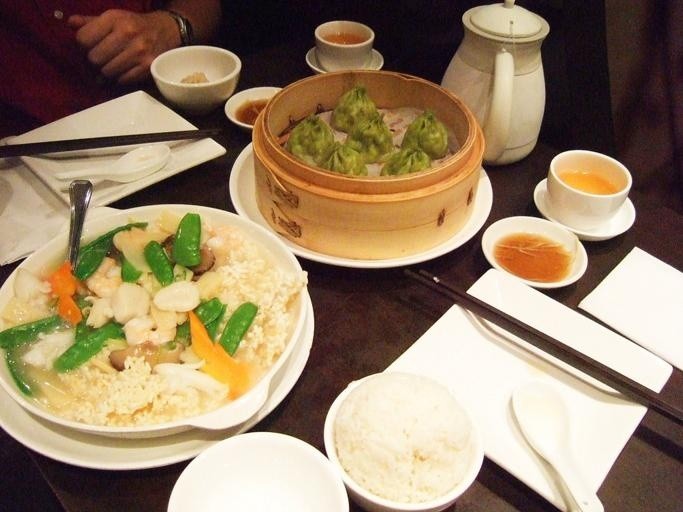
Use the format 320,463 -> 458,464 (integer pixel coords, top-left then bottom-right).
0,0 -> 223,138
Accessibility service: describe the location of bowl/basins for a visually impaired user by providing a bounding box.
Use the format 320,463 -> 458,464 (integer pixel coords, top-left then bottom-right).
149,43 -> 242,112
165,430 -> 349,511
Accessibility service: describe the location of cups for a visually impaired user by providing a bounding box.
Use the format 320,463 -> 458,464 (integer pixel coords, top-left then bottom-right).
313,19 -> 375,75
544,150 -> 635,230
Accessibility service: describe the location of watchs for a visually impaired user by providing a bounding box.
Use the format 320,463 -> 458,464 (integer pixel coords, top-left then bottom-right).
162,7 -> 193,46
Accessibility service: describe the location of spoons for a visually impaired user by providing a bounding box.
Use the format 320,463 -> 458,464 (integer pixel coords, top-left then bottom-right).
507,379 -> 602,512
56,138 -> 170,187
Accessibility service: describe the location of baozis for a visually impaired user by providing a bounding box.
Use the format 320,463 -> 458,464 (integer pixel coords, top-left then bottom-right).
284,84 -> 447,178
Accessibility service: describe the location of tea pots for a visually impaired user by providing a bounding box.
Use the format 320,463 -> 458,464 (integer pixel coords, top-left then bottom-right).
442,1 -> 551,169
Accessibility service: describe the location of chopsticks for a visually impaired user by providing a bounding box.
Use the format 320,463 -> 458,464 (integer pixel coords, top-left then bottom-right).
1,125 -> 226,159
401,265 -> 681,425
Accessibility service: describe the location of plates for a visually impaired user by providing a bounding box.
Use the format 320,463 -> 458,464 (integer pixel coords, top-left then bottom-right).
22,90 -> 225,208
0,156 -> 77,265
381,266 -> 673,509
479,216 -> 588,290
227,135 -> 491,272
302,43 -> 386,75
228,84 -> 281,131
532,177 -> 636,246
1,281 -> 317,471
